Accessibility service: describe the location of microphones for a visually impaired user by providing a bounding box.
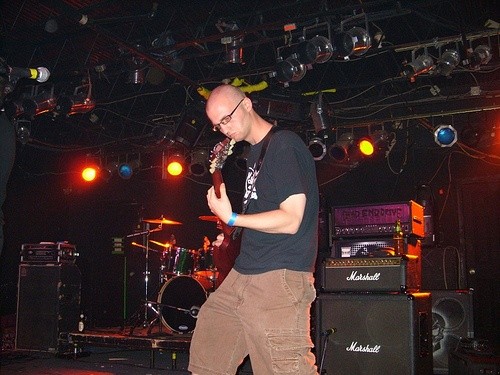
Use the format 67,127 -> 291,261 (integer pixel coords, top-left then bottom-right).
191,309 -> 198,314
321,328 -> 337,336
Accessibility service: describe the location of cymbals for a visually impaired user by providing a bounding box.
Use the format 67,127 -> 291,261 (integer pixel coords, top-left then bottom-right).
199,216 -> 220,221
141,218 -> 182,224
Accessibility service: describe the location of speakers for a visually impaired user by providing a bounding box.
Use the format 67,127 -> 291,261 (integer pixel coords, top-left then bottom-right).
14,264 -> 83,352
314,288 -> 474,375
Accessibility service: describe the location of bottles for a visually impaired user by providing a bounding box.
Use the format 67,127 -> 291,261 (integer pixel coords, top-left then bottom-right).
78,311 -> 85,332
392,219 -> 405,257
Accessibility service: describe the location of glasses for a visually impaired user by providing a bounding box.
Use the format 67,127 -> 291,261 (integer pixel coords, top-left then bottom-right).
212,99 -> 243,132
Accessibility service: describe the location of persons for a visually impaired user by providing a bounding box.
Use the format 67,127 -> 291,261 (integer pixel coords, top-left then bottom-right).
199,234 -> 215,270
188,85 -> 320,375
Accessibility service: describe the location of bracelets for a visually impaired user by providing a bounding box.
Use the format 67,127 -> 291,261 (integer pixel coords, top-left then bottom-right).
227,212 -> 237,226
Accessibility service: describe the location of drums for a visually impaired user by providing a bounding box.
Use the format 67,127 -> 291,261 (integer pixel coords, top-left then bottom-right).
172,247 -> 202,275
157,274 -> 215,335
196,247 -> 219,279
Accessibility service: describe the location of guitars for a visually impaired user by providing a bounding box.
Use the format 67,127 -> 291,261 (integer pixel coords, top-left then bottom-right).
209,138 -> 241,290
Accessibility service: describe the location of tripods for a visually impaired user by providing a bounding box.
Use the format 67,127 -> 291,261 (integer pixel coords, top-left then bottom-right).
127,222 -> 163,328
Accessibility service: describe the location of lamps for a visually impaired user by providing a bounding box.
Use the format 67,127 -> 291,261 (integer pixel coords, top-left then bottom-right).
400,45 -> 494,82
358,128 -> 388,158
328,130 -> 355,162
188,144 -> 211,177
116,151 -> 143,180
165,148 -> 186,177
275,11 -> 373,85
63,77 -> 97,117
130,58 -> 144,92
18,83 -> 57,118
222,31 -> 243,68
434,121 -> 457,148
79,152 -> 101,183
307,134 -> 326,163
